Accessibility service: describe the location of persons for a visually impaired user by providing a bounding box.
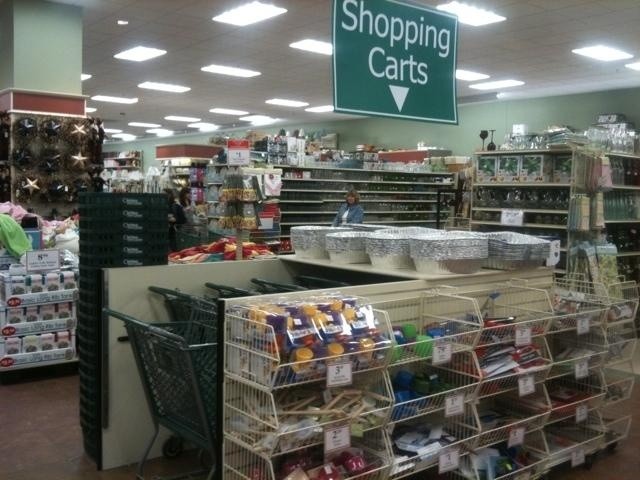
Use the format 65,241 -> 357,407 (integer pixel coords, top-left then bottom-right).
332,189 -> 364,227
178,187 -> 204,224
161,188 -> 186,223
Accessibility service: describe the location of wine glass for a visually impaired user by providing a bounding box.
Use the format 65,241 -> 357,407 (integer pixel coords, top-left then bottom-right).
480,129 -> 496,151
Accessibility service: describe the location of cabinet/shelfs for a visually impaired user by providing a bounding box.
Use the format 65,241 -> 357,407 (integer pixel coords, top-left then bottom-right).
218,270 -> 640,480
475,147 -> 640,300
101,151 -> 143,174
159,161 -> 459,249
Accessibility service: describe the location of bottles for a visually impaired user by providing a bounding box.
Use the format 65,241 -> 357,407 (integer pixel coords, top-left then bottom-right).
604,156 -> 640,253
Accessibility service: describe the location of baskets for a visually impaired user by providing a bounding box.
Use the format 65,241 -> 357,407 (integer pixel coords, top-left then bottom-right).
76,191 -> 168,457
